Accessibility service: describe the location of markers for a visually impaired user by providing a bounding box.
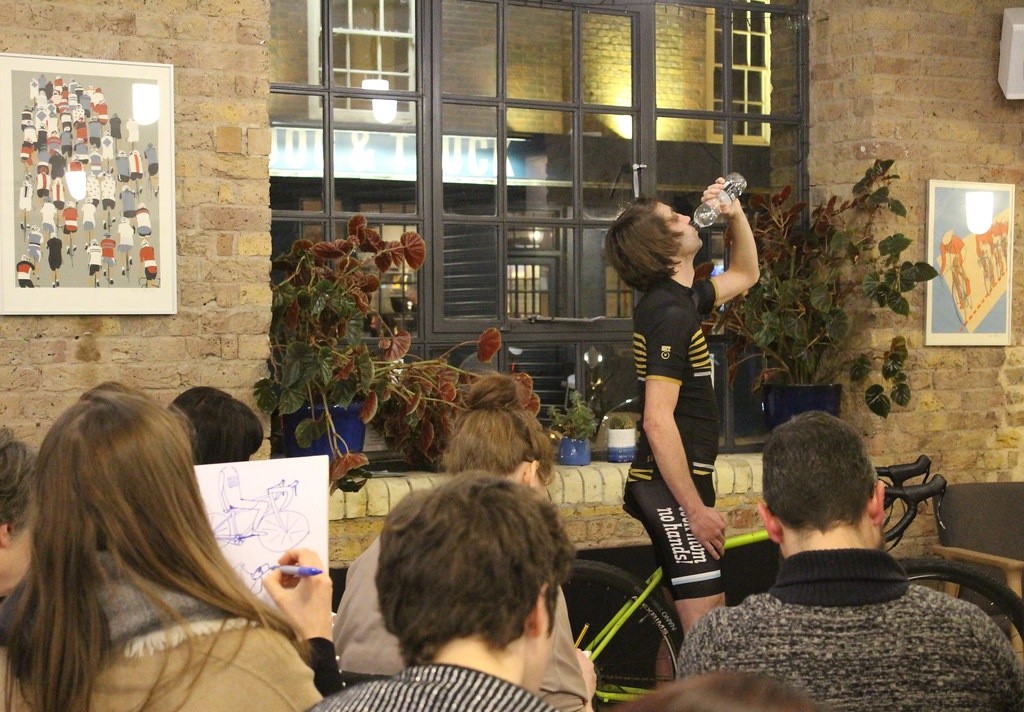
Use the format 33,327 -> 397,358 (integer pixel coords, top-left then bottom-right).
270,565 -> 323,576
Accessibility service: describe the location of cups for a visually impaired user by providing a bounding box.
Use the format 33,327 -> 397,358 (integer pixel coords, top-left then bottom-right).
608,429 -> 636,462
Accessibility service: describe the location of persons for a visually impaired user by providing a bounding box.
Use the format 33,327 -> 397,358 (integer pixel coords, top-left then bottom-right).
675,410 -> 1023,712
0,374 -> 341,712
604,177 -> 759,688
330,372 -> 597,712
300,473 -> 574,712
606,670 -> 814,711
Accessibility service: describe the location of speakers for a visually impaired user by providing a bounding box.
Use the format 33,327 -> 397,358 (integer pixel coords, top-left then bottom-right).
997,7 -> 1024,100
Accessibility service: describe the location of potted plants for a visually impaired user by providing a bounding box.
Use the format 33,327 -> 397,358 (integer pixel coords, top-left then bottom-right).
693,158 -> 939,436
604,415 -> 637,463
252,212 -> 542,501
546,390 -> 599,466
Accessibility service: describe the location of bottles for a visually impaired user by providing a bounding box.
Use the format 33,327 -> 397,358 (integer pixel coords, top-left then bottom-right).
688,173 -> 748,232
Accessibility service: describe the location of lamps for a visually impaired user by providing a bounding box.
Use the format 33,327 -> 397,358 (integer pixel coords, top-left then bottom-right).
995,7 -> 1024,101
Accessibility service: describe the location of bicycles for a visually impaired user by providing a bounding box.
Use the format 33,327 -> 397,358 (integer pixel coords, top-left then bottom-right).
562,455 -> 1024,703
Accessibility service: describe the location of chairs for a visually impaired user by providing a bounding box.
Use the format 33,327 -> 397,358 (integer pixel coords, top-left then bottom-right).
931,481 -> 1024,667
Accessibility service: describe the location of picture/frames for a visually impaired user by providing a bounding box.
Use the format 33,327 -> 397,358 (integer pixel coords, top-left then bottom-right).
923,178 -> 1016,347
0,52 -> 179,319
